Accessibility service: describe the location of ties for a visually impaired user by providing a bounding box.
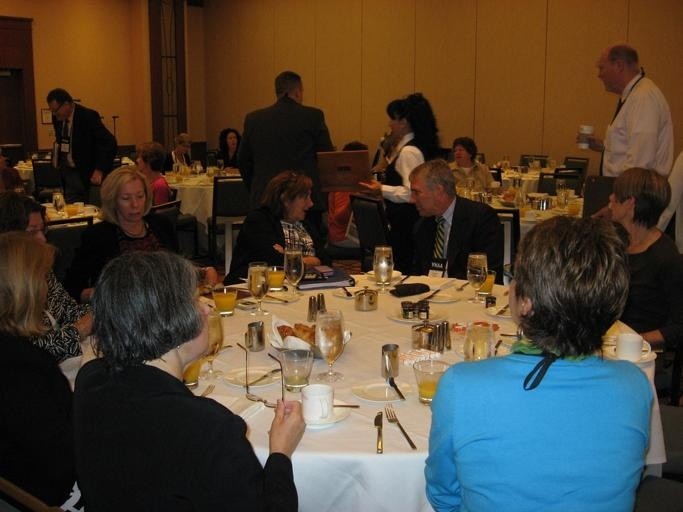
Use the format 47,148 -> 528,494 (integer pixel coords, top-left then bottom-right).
60,118 -> 70,161
431,216 -> 446,258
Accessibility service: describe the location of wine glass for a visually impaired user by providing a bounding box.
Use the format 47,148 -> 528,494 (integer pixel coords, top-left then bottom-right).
314,310 -> 346,382
465,253 -> 488,304
246,261 -> 270,317
283,247 -> 304,297
456,158 -> 589,219
373,247 -> 393,295
201,315 -> 226,380
463,323 -> 495,365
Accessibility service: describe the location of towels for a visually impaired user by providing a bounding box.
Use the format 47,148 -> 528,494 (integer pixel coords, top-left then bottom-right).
204,394 -> 265,419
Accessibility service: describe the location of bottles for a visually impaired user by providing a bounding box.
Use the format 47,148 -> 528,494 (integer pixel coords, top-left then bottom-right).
420,320 -> 452,354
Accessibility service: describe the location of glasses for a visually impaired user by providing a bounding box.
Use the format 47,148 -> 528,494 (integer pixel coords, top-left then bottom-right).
24,224 -> 49,237
48,103 -> 63,114
502,264 -> 515,280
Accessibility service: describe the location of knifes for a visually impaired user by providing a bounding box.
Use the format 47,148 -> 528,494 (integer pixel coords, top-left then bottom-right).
244,368 -> 282,388
374,411 -> 383,454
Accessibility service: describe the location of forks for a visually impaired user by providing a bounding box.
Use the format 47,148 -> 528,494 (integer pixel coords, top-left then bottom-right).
384,403 -> 417,450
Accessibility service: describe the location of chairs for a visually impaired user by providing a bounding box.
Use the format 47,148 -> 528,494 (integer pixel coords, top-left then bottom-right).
655,405 -> 682,476
634,475 -> 682,511
0,477 -> 63,511
0,141 -> 251,284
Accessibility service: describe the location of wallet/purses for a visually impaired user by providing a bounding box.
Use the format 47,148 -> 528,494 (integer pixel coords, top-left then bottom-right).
389,283 -> 430,297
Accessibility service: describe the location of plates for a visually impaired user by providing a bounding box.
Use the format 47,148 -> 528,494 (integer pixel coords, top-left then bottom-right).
298,398 -> 355,432
262,296 -> 300,305
366,270 -> 403,280
386,311 -> 449,324
225,366 -> 281,391
606,346 -> 658,365
44,189 -> 101,232
335,286 -> 364,299
352,378 -> 413,402
421,293 -> 459,304
485,306 -> 511,319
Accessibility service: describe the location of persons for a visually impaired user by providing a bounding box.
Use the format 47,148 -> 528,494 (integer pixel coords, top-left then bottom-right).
222,171 -> 333,285
61,165 -> 218,301
74,247 -> 306,512
1,72 -> 494,247
425,215 -> 653,512
575,44 -> 674,219
609,167 -> 682,344
1,193 -> 93,364
0,231 -> 86,512
401,158 -> 504,284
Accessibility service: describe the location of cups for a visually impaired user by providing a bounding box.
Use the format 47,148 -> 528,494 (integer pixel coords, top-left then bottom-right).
210,288 -> 238,317
300,383 -> 336,421
268,266 -> 286,292
485,271 -> 496,292
412,359 -> 449,404
614,334 -> 652,361
183,361 -> 201,389
164,158 -> 244,188
381,342 -> 399,379
576,123 -> 594,150
277,350 -> 314,393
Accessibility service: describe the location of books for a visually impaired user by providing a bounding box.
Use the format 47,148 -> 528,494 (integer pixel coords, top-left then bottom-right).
297,266 -> 356,289
